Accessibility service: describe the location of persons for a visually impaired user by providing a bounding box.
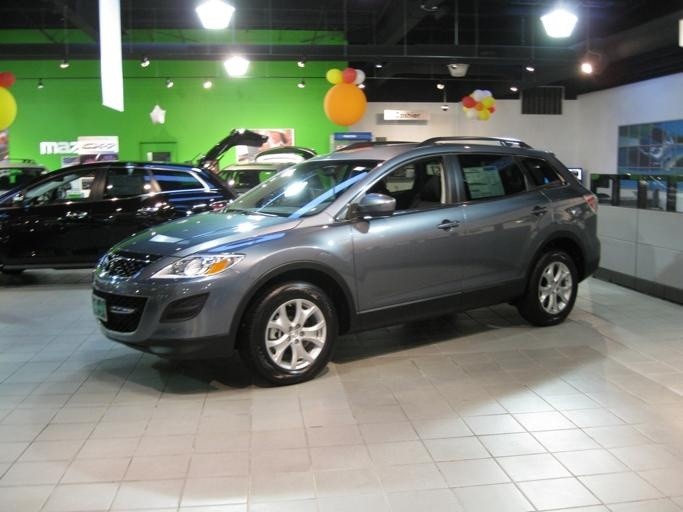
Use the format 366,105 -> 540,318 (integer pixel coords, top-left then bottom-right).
16,169 -> 34,185
0,176 -> 12,195
104,170 -> 131,199
247,128 -> 293,162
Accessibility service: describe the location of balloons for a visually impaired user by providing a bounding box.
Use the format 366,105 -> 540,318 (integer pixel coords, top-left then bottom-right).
355,70 -> 365,84
463,89 -> 497,120
0,72 -> 15,87
324,83 -> 367,125
342,68 -> 356,83
0,88 -> 16,131
326,68 -> 342,84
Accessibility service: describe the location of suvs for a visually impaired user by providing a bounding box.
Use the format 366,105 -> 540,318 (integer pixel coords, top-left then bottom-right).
90,136 -> 601,385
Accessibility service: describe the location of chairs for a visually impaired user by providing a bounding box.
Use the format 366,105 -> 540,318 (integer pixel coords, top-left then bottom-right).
349,170 -> 441,209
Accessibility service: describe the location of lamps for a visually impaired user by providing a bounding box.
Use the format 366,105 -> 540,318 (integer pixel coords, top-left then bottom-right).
358,82 -> 366,90
376,61 -> 383,68
223,0 -> 250,78
297,56 -> 307,67
297,80 -> 306,89
60,0 -> 70,70
165,78 -> 174,89
203,80 -> 213,88
37,78 -> 45,90
525,18 -> 536,72
446,0 -> 470,78
509,82 -> 520,93
436,80 -> 446,90
141,56 -> 150,67
